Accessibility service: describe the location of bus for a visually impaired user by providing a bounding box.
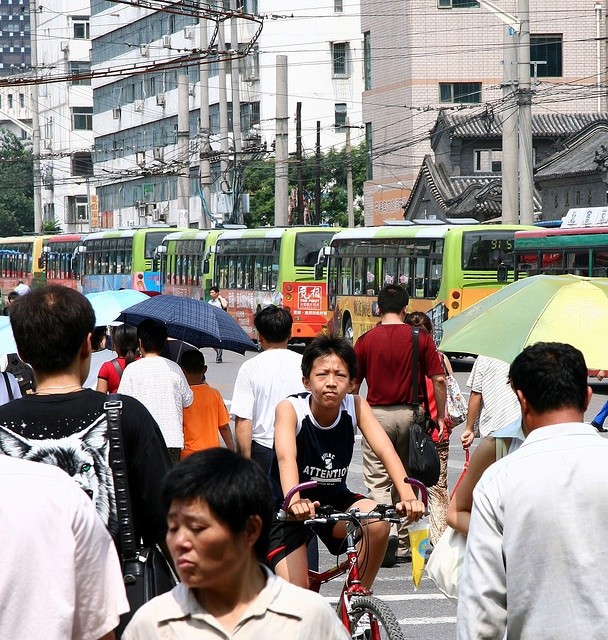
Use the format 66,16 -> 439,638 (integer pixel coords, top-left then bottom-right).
203,225 -> 340,345
498,207 -> 608,385
315,218 -> 545,358
0,235 -> 53,315
38,234 -> 88,293
70,225 -> 188,297
152,229 -> 223,301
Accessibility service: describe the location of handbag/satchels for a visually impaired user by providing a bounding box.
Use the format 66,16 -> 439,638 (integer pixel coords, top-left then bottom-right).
408,323 -> 441,487
104,394 -> 176,640
441,353 -> 468,429
425,437 -> 508,606
218,298 -> 227,312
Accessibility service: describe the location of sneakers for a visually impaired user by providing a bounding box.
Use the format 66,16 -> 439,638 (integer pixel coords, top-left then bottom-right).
381,535 -> 399,568
397,556 -> 412,564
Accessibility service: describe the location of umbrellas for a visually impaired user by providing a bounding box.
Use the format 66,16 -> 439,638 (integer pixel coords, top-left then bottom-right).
74,287 -> 152,346
113,291 -> 258,377
436,273 -> 608,370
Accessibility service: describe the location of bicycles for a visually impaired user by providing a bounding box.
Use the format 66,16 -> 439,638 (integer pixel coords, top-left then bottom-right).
277,477 -> 431,640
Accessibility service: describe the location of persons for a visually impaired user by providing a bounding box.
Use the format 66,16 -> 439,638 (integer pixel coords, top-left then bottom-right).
16,278 -> 31,298
3,289 -> 20,316
265,330 -> 424,609
176,345 -> 235,461
341,283 -> 449,569
405,305 -> 467,553
443,409 -> 531,535
461,348 -> 523,451
206,284 -> 231,367
1,372 -> 22,405
80,321 -> 118,394
117,319 -> 194,472
94,323 -> 142,394
592,386 -> 608,433
231,303 -> 320,481
0,284 -> 173,599
121,446 -> 355,639
456,343 -> 606,640
1,449 -> 132,640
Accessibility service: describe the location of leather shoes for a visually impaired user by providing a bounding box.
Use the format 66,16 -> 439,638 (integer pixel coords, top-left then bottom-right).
591,420 -> 607,432
